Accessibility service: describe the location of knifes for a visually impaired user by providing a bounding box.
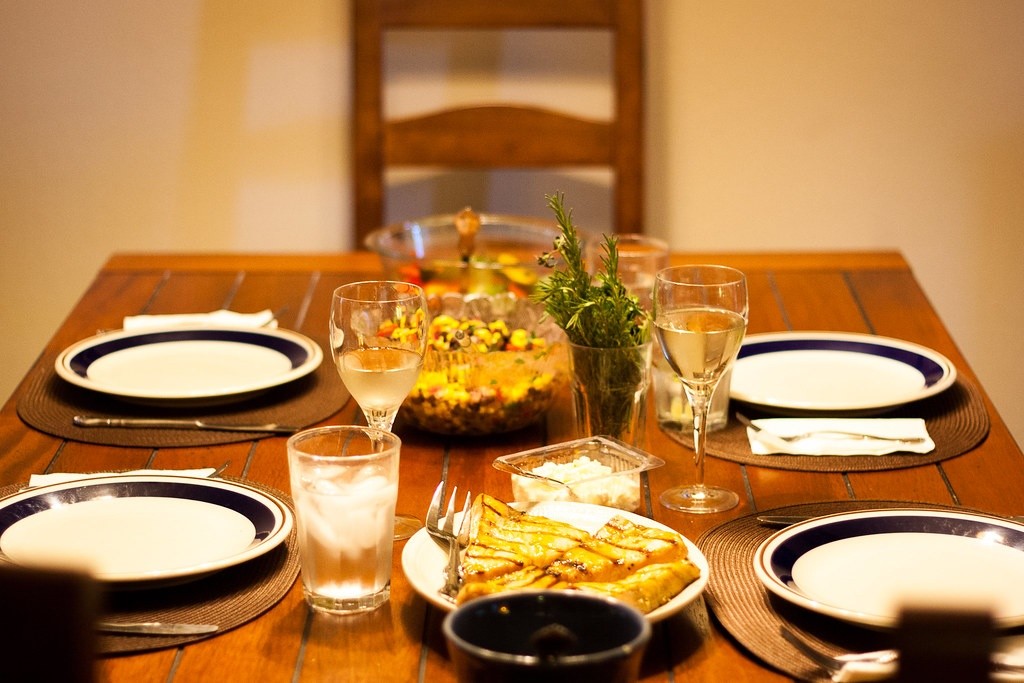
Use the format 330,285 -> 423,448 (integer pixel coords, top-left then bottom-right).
73,415 -> 301,432
756,515 -> 819,526
94,621 -> 219,634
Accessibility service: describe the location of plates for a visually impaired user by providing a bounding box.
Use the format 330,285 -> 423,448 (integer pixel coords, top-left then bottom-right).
0,475 -> 293,582
401,500 -> 708,625
729,330 -> 958,410
55,322 -> 324,399
754,508 -> 1024,630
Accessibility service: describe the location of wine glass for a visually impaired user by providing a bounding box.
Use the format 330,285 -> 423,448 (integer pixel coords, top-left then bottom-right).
653,264 -> 749,514
329,281 -> 423,540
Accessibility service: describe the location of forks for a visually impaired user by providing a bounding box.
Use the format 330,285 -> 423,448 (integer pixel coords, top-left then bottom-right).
779,625 -> 843,672
209,460 -> 231,477
737,412 -> 925,446
426,481 -> 473,596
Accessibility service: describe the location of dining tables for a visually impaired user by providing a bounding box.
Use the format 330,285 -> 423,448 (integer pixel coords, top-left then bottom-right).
0,248 -> 1024,683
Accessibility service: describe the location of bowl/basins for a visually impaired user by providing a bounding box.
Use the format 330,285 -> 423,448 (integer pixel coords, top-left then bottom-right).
496,437 -> 664,514
392,298 -> 570,436
361,211 -> 581,297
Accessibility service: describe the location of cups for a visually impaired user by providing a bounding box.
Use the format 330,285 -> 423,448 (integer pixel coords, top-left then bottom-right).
286,426 -> 401,616
652,336 -> 735,432
584,234 -> 669,346
444,590 -> 650,683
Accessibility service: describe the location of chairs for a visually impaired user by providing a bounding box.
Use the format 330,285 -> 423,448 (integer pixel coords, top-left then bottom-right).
346,0 -> 644,252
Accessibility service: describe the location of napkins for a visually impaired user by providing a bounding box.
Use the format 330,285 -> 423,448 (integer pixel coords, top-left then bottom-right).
125,310 -> 276,332
30,468 -> 217,487
744,417 -> 937,456
